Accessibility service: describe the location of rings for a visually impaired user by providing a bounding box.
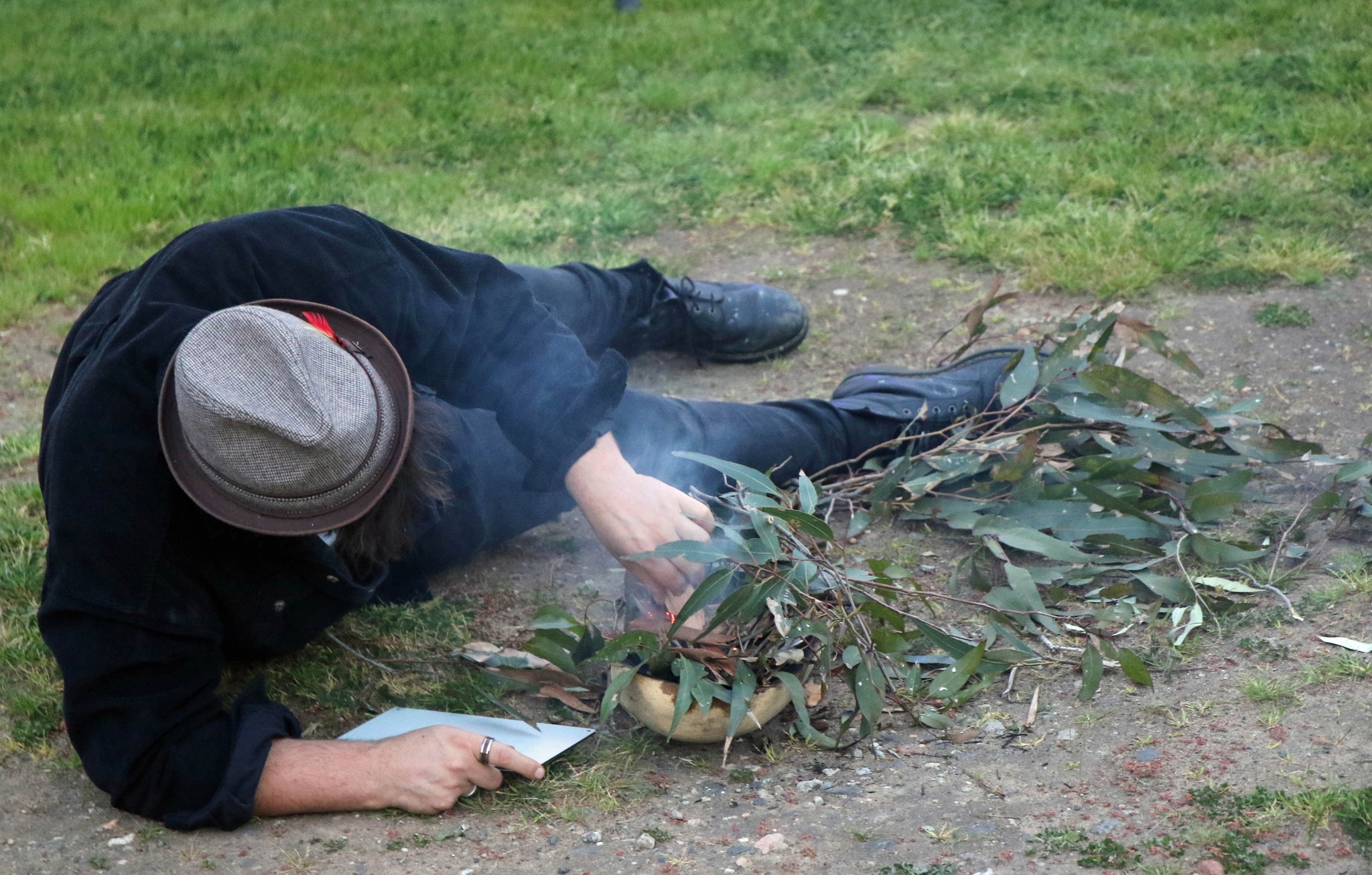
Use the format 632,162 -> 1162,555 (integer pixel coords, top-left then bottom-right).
462,785 -> 478,798
480,736 -> 495,765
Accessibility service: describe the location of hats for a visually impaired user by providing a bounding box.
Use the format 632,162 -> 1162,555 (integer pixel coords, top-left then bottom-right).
157,295 -> 415,537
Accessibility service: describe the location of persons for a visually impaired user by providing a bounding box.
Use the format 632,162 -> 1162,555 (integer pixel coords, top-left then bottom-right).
35,204 -> 1079,834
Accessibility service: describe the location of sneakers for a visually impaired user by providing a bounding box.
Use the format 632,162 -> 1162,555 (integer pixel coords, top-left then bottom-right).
614,259 -> 810,362
830,347 -> 1079,456
